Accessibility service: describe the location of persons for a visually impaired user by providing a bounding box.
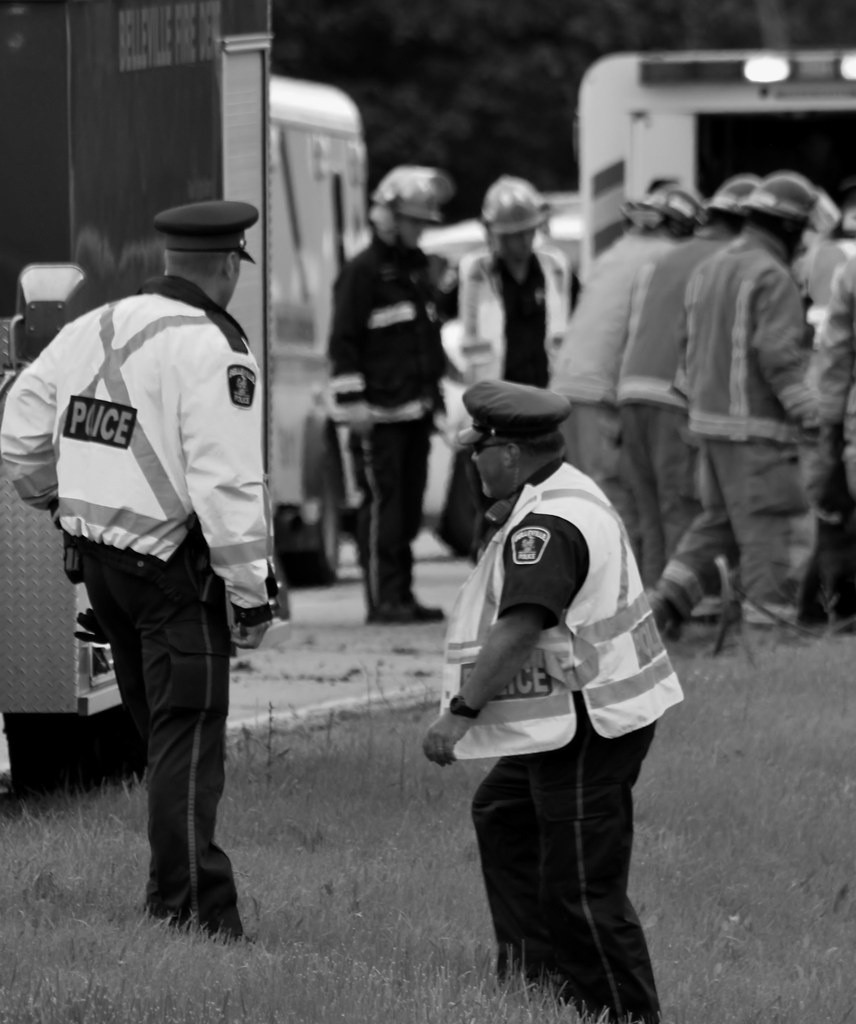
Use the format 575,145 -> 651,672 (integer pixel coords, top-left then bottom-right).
415,381 -> 684,1024
0,201 -> 278,935
322,164 -> 855,633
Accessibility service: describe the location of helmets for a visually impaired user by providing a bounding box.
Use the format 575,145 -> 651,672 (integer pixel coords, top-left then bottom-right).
480,175 -> 552,236
371,165 -> 453,224
710,176 -> 816,223
624,178 -> 700,233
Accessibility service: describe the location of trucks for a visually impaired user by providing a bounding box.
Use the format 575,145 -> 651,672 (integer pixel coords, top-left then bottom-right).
0,0 -> 274,798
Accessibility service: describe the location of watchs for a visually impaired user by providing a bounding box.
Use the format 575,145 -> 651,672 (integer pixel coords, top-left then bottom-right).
449,695 -> 480,719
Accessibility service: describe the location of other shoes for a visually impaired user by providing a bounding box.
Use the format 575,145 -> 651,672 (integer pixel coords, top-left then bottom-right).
151,899 -> 241,939
650,589 -> 687,642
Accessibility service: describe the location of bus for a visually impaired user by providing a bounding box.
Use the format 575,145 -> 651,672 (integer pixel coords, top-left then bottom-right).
271,74 -> 373,587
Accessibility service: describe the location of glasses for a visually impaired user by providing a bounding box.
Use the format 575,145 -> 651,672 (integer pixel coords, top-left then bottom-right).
471,441 -> 509,456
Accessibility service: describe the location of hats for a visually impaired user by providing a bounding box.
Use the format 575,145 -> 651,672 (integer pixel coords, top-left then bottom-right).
458,379 -> 571,447
156,201 -> 260,263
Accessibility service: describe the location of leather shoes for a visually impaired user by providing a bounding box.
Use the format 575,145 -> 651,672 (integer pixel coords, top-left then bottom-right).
362,602 -> 445,625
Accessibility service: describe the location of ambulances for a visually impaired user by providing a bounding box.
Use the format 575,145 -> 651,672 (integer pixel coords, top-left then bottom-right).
574,48 -> 856,289
